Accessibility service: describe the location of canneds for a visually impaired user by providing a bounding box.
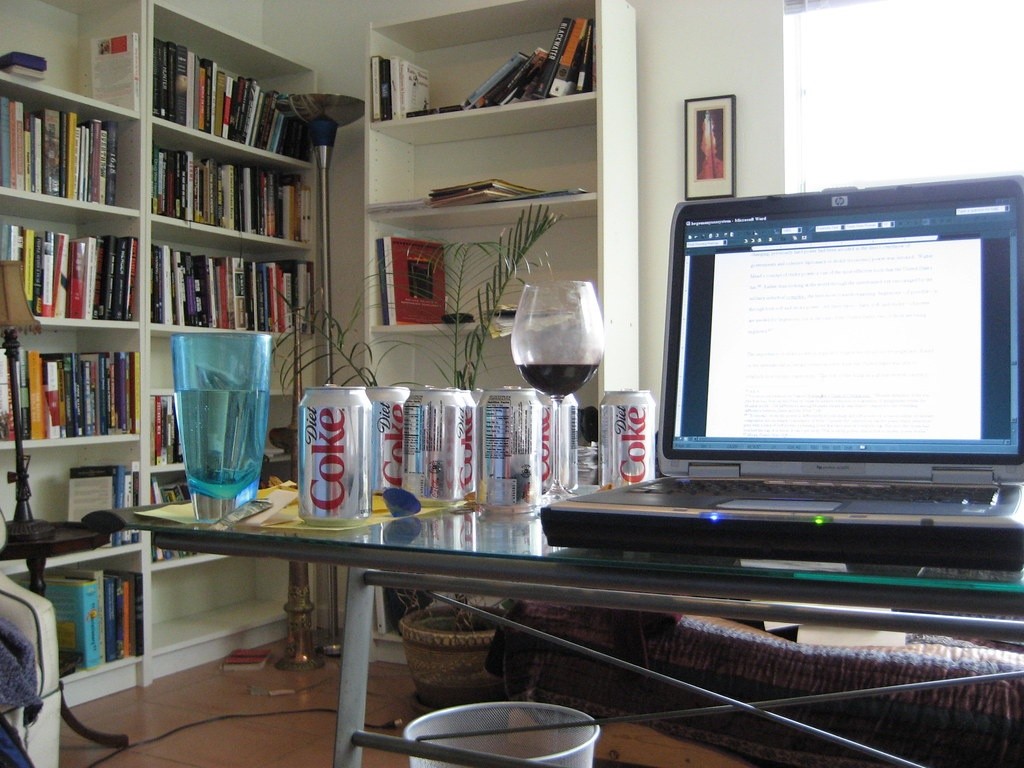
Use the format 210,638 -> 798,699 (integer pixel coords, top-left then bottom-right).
298,385 -> 655,528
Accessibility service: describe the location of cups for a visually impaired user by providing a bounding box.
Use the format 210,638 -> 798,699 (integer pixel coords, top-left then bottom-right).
171,332 -> 272,523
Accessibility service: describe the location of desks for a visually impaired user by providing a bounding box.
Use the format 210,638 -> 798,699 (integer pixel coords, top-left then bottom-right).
0,522 -> 129,747
83,483 -> 1023,768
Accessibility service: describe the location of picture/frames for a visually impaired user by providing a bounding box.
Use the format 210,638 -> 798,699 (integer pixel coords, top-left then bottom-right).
684,95 -> 737,199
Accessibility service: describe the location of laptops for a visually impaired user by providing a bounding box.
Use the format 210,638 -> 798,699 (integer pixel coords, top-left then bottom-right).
541,176 -> 1024,573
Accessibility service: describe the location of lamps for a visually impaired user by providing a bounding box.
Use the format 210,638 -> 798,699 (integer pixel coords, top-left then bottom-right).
273,257 -> 324,671
275,94 -> 363,656
0,260 -> 57,540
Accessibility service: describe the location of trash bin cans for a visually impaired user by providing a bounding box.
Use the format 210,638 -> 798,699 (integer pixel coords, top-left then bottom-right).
403,701 -> 601,768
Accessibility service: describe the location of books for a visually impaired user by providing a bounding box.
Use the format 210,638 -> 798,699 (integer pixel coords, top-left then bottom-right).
376,236 -> 446,325
1,38 -> 312,670
367,178 -> 586,213
371,17 -> 596,122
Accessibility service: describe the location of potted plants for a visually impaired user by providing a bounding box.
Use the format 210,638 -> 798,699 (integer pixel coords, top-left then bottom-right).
269,204 -> 564,707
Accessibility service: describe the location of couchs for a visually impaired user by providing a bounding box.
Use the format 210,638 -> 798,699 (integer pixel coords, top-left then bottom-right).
0,507 -> 61,768
484,547 -> 1024,768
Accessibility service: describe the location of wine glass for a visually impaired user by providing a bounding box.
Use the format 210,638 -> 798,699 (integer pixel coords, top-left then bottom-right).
510,281 -> 603,516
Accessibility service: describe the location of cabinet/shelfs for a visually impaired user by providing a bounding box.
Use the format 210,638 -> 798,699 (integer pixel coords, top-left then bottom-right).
0,0 -> 316,709
363,0 -> 639,485
